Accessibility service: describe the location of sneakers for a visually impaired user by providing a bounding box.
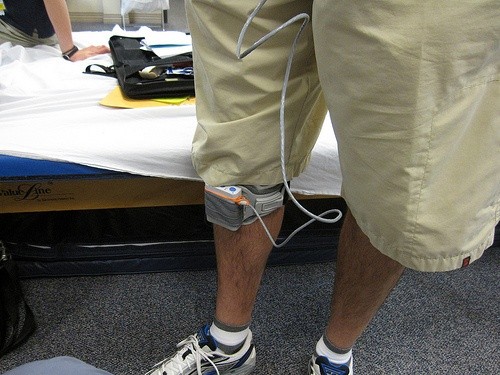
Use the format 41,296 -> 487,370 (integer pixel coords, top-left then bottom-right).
136,325 -> 258,375
304,353 -> 354,375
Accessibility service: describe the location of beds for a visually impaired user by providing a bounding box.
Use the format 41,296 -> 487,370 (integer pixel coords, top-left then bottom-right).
0,32 -> 348,279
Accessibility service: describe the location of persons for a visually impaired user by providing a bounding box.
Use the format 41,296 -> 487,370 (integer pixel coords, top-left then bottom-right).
0,0 -> 110,63
142,0 -> 500,375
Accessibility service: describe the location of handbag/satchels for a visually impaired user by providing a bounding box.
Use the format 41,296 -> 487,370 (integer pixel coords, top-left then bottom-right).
83,33 -> 195,99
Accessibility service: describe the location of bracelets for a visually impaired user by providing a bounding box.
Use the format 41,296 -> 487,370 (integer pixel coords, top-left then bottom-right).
63,46 -> 78,59
